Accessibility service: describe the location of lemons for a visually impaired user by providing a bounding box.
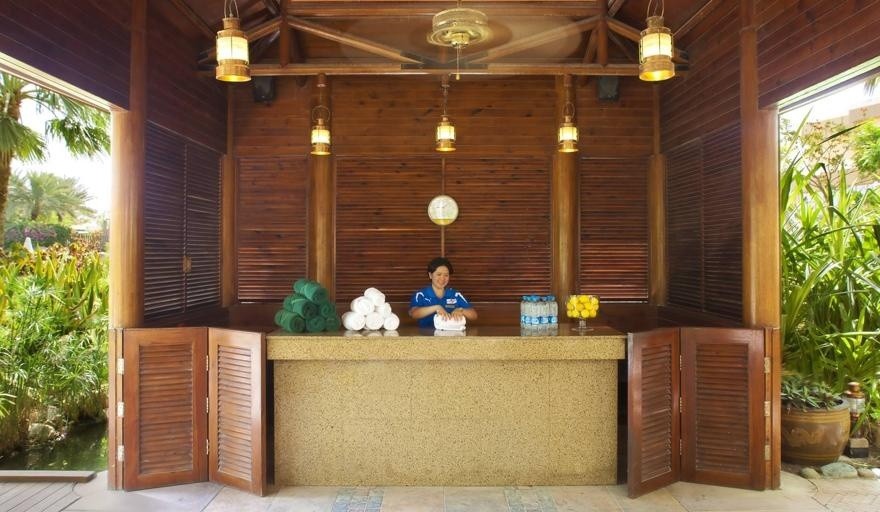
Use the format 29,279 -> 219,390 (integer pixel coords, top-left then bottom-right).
565,295 -> 599,319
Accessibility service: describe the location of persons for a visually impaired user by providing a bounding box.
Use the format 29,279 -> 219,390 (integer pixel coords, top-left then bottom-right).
407,256 -> 479,327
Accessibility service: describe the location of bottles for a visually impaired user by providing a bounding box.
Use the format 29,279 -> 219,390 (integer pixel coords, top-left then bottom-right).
520,295 -> 559,326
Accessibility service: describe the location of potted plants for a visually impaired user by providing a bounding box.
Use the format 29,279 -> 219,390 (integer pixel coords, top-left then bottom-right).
780,375 -> 851,467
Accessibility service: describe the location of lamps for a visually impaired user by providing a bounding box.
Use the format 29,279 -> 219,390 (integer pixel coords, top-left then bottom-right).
557,74 -> 580,153
426,0 -> 492,82
214,0 -> 252,82
310,72 -> 332,155
638,0 -> 675,82
434,75 -> 457,152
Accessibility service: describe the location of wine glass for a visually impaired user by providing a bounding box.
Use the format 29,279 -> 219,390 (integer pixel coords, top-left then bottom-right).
564,295 -> 601,332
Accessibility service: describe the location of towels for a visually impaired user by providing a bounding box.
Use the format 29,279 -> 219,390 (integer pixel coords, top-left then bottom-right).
343,331 -> 400,338
273,281 -> 400,333
433,328 -> 466,337
433,313 -> 467,331
